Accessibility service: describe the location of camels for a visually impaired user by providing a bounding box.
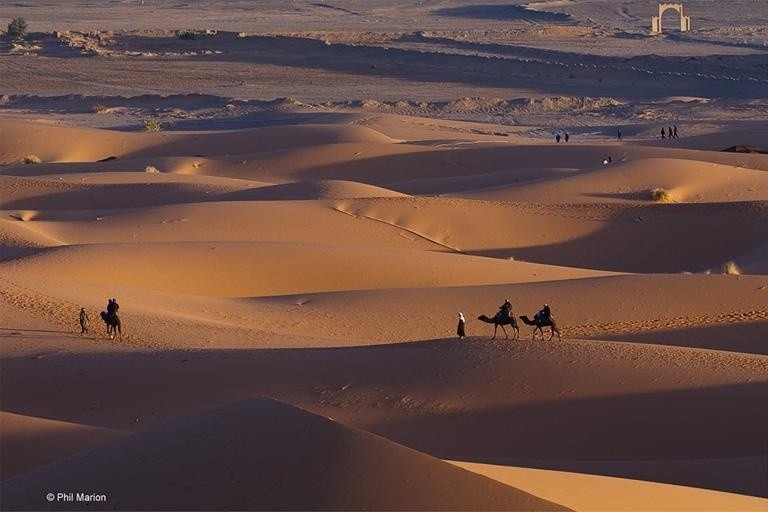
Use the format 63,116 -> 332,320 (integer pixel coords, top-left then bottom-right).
100,311 -> 122,342
519,312 -> 561,342
477,309 -> 519,341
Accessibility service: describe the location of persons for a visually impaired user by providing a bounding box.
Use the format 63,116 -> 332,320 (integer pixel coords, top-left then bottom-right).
498,299 -> 512,323
103,299 -> 112,324
538,303 -> 549,323
667,126 -> 673,139
617,128 -> 622,142
106,299 -> 119,323
672,124 -> 677,138
660,127 -> 665,140
555,133 -> 560,144
456,312 -> 465,339
79,308 -> 90,334
563,133 -> 568,144
